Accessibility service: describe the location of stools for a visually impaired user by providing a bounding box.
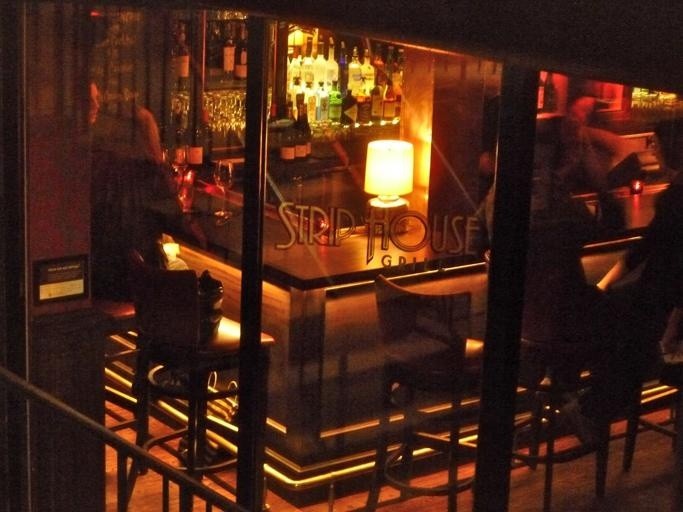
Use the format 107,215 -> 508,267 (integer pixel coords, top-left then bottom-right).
98,300 -> 154,475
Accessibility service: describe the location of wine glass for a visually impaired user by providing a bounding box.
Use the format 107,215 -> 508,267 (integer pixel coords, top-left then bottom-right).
214,159 -> 234,217
175,90 -> 246,132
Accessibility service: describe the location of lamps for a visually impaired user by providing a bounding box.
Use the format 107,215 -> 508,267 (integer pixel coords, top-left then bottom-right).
364,139 -> 413,202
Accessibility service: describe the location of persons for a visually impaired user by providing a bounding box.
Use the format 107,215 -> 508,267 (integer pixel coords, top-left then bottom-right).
91,102 -> 179,302
87,79 -> 101,176
595,116 -> 682,372
472,113 -> 631,448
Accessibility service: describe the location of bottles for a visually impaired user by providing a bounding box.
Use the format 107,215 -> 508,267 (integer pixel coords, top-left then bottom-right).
219,17 -> 247,80
287,77 -> 397,123
171,22 -> 190,88
172,109 -> 205,173
288,36 -> 376,89
279,101 -> 311,162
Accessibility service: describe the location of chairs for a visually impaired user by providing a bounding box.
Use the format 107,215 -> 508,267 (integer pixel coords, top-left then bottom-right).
607,244 -> 682,471
126,250 -> 275,511
511,265 -> 633,512
365,275 -> 485,511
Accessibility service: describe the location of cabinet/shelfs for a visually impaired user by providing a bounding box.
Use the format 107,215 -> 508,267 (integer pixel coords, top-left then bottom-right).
148,4 -> 405,152
